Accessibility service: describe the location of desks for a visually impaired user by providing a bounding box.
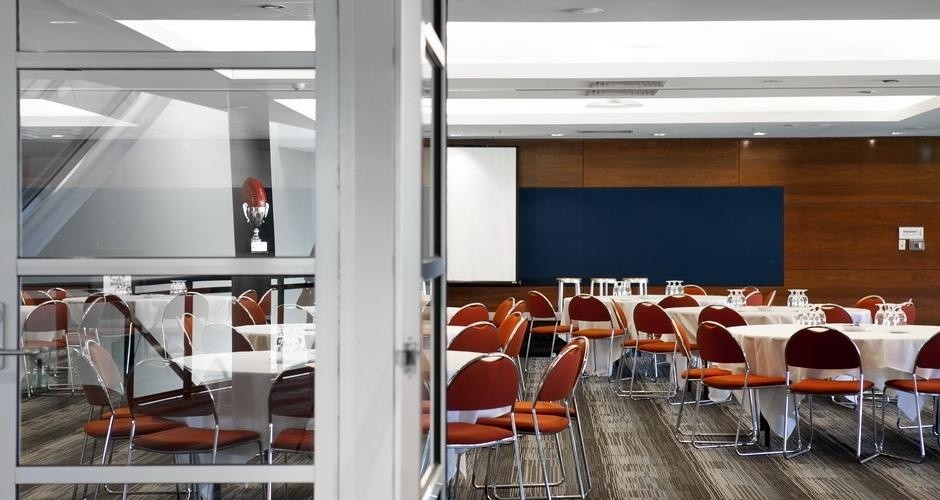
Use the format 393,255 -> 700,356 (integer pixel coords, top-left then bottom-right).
60,293 -> 182,336
563,294 -> 727,376
707,325 -> 940,441
136,349 -> 315,498
446,350 -> 515,491
664,306 -> 873,405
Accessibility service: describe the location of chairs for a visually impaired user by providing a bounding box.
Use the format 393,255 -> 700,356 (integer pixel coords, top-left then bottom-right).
898,301 -> 916,324
497,310 -> 523,351
445,354 -> 526,499
176,313 -> 200,350
82,292 -> 104,314
238,289 -> 258,302
612,300 -> 664,397
763,289 -> 776,305
744,291 -> 762,306
879,330 -> 940,463
524,290 -> 578,372
671,318 -> 753,444
741,286 -> 759,297
47,288 -> 71,303
629,302 -> 700,400
238,296 -> 266,325
257,288 -> 274,315
692,320 -> 802,455
504,300 -> 526,318
71,349 -> 194,499
85,341 -> 151,497
492,297 -> 515,328
446,321 -> 499,354
265,368 -> 314,498
569,293 -> 625,382
67,297 -> 106,379
20,288 -> 55,306
200,322 -> 256,353
448,302 -> 489,326
854,294 -> 886,321
818,304 -> 852,323
783,326 -> 880,465
272,304 -> 314,322
153,292 -> 210,358
698,304 -> 750,328
122,358 -> 265,499
95,295 -> 135,373
20,302 -> 75,398
658,294 -> 699,307
498,315 -> 528,392
682,285 -> 707,295
228,299 -> 258,327
472,336 -> 592,489
485,346 -> 585,497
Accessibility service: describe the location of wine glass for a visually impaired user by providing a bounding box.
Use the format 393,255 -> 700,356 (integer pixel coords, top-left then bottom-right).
874,303 -> 908,328
725,288 -> 746,306
110,275 -> 132,299
786,289 -> 808,308
613,280 -> 632,297
169,280 -> 188,296
796,303 -> 826,328
665,280 -> 684,295
